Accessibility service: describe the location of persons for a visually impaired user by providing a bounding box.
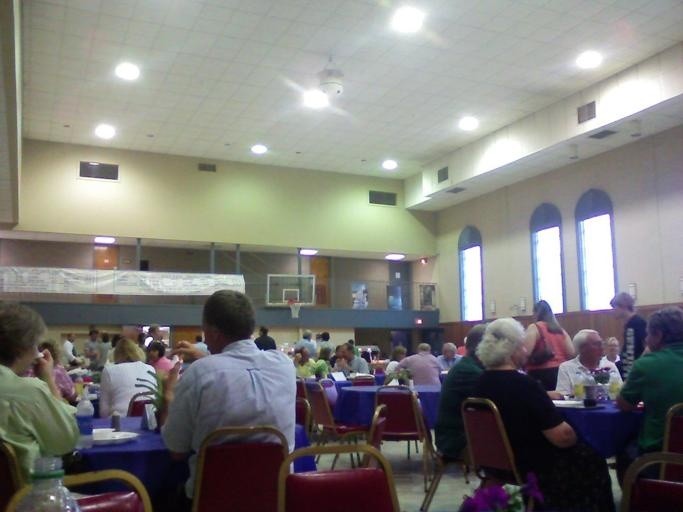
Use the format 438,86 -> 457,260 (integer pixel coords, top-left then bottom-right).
0,301 -> 79,485
471,315 -> 582,511
436,342 -> 463,372
98,337 -> 158,417
615,305 -> 683,491
385,345 -> 407,386
254,326 -> 277,352
148,341 -> 180,380
289,327 -> 370,378
62,332 -> 84,368
556,328 -> 624,511
22,340 -> 78,405
434,323 -> 485,463
519,300 -> 575,391
84,329 -> 99,363
395,343 -> 442,386
602,337 -> 620,363
610,292 -> 646,380
192,334 -> 208,352
99,333 -> 111,361
106,334 -> 122,362
161,289 -> 297,512
147,323 -> 168,349
457,336 -> 468,355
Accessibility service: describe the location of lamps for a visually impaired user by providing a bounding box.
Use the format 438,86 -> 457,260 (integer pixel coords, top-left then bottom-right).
315,56 -> 344,96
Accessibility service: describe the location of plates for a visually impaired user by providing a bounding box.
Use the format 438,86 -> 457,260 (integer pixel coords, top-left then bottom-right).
93,433 -> 139,445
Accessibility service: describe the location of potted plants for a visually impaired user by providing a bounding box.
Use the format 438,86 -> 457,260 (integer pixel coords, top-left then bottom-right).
310,360 -> 329,381
135,370 -> 163,432
595,373 -> 610,398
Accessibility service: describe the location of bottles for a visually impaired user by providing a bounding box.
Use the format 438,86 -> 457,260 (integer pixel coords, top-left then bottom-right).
572,370 -> 586,399
4,456 -> 78,512
73,388 -> 97,450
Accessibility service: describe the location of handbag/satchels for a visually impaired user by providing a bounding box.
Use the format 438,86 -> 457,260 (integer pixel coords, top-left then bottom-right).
527,323 -> 554,365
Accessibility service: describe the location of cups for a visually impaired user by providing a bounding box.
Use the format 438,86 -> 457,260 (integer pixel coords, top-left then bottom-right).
584,384 -> 597,399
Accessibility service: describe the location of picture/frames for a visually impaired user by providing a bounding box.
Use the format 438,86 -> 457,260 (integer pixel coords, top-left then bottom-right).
416,282 -> 439,311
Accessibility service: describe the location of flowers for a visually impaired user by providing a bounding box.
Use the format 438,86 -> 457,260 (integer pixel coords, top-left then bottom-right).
458,472 -> 543,512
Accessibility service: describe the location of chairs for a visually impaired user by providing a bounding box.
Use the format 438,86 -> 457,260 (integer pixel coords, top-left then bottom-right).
192,426 -> 289,512
319,378 -> 338,410
295,398 -> 311,435
128,391 -> 154,417
410,390 -> 463,512
0,441 -> 24,512
277,445 -> 400,512
461,396 -> 533,512
6,469 -> 152,512
620,452 -> 683,512
375,385 -> 446,499
352,374 -> 375,387
296,376 -> 307,399
310,381 -> 367,471
659,403 -> 683,482
